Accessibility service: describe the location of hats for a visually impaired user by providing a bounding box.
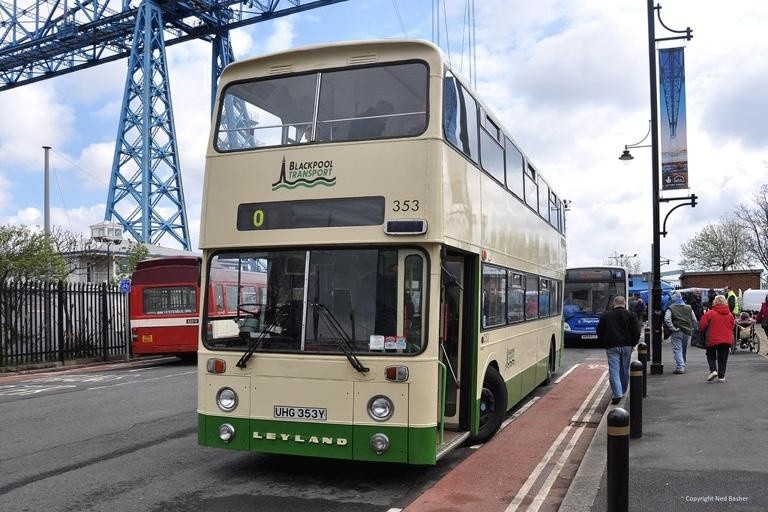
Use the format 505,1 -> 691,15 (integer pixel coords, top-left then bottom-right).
671,293 -> 683,302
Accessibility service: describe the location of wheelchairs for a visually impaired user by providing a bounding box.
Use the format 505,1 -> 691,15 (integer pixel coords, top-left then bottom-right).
728,320 -> 761,355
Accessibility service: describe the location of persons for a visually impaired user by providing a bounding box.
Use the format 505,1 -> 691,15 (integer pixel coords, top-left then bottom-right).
756,294 -> 767,356
732,312 -> 755,351
662,292 -> 696,374
698,295 -> 735,383
595,295 -> 641,405
686,286 -> 738,347
629,294 -> 647,321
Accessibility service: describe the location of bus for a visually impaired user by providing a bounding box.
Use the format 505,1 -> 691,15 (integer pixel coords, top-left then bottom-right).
564,266 -> 633,347
195,35 -> 572,469
129,250 -> 267,358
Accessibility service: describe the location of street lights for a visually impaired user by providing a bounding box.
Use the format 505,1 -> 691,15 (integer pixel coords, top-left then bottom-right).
617,139 -> 664,375
94,236 -> 122,289
620,254 -> 637,266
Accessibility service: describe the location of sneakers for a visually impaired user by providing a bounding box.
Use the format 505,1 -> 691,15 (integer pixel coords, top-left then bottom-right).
611,397 -> 622,405
672,367 -> 685,374
718,377 -> 725,381
706,371 -> 718,382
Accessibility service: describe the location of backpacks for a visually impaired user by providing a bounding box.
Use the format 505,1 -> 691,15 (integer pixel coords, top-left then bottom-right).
739,327 -> 751,339
